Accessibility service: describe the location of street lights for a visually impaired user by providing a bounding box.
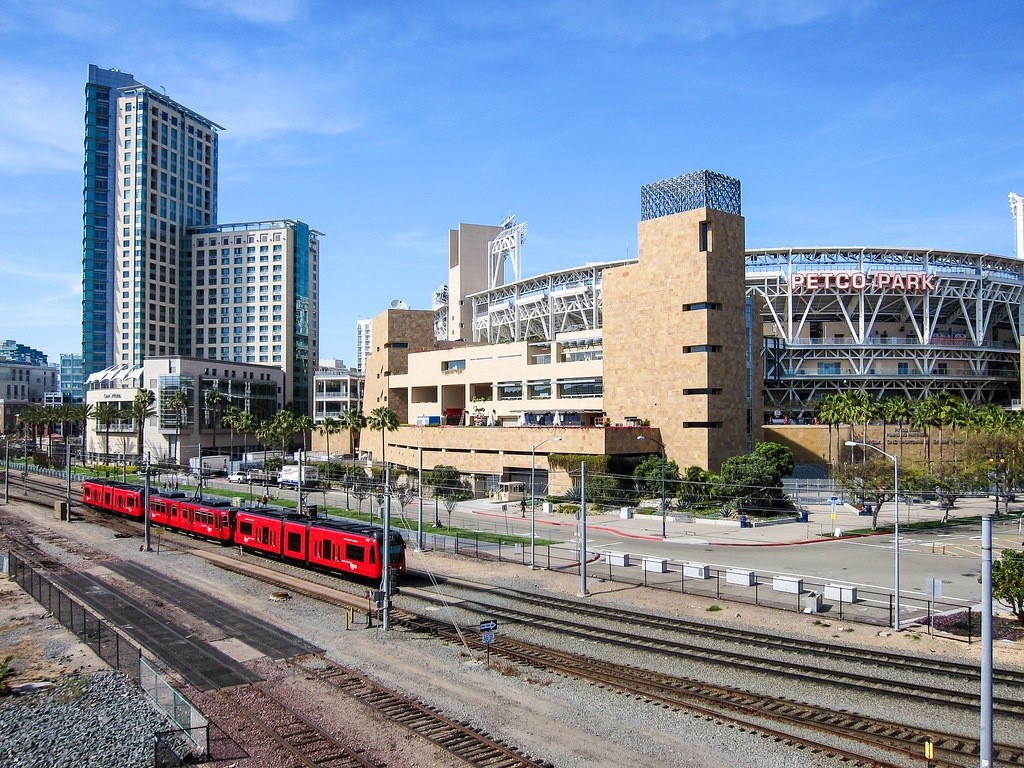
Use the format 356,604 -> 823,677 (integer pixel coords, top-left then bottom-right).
988,452 -> 1006,518
637,434 -> 668,539
845,437 -> 901,632
532,436 -> 563,570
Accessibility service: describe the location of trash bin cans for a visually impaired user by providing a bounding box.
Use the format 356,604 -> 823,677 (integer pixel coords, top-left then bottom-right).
741,516 -> 747,528
866,504 -> 872,516
802,512 -> 808,522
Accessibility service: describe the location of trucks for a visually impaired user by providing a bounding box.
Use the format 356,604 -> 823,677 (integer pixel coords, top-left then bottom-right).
242,450 -> 287,463
277,465 -> 319,491
245,469 -> 278,486
293,451 -> 327,463
189,454 -> 229,479
1001,399 -> 1021,411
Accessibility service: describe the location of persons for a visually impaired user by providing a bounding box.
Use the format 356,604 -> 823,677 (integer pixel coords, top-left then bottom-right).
491,409 -> 497,426
255,496 -> 260,507
162,482 -> 166,492
174,481 -> 178,491
167,480 -> 171,491
262,495 -> 268,507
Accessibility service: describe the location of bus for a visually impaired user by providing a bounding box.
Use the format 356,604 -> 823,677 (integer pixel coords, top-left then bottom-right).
796,408 -> 820,424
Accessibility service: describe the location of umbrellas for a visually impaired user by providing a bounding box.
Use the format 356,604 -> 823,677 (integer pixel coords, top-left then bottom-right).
520,497 -> 527,518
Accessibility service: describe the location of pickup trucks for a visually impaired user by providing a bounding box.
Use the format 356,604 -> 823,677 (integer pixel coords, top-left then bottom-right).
226,471 -> 249,484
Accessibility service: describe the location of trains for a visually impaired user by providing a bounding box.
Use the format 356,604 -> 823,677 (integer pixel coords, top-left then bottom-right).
79,478 -> 407,583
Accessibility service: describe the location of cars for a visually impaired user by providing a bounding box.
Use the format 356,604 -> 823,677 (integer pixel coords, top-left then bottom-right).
826,497 -> 845,505
284,453 -> 296,460
768,415 -> 793,424
